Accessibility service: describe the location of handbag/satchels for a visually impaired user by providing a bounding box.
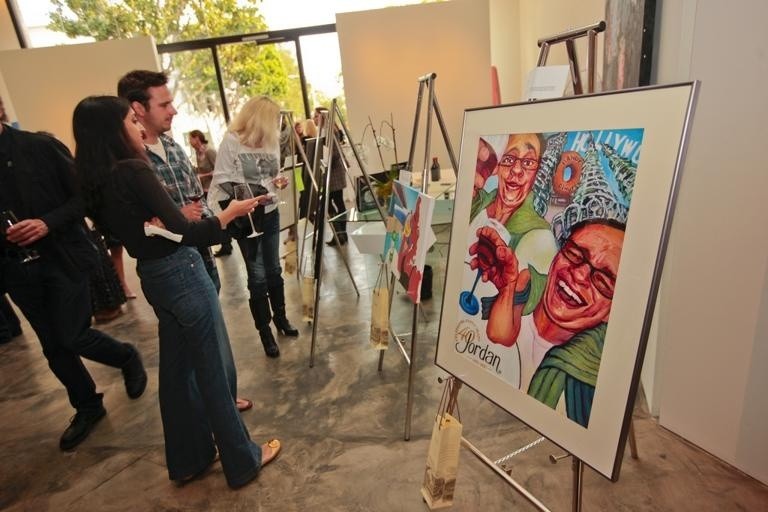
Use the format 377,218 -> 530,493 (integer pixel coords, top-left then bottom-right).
422,413 -> 463,510
302,277 -> 315,323
370,287 -> 391,350
284,235 -> 297,275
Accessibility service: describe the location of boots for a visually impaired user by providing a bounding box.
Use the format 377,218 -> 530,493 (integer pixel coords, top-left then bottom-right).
270,287 -> 298,336
250,297 -> 280,357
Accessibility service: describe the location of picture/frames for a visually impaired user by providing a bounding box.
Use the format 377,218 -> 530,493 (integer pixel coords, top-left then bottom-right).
434,77 -> 704,485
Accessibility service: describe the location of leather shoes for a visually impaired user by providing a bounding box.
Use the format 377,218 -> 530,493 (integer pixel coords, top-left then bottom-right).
119,343 -> 147,398
60,409 -> 105,449
257,439 -> 280,466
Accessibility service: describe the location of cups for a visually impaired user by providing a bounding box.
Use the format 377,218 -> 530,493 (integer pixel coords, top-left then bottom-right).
473,138 -> 498,189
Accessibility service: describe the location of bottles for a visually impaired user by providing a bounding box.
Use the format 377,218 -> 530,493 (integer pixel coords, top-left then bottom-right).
388,230 -> 396,262
430,157 -> 441,182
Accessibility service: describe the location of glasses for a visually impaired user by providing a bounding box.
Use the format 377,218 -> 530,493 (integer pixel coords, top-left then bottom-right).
498,156 -> 542,171
560,237 -> 616,300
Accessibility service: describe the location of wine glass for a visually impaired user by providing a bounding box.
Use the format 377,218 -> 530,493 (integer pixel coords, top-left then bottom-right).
273,167 -> 286,206
187,178 -> 202,204
3,211 -> 40,266
458,218 -> 506,316
233,184 -> 265,239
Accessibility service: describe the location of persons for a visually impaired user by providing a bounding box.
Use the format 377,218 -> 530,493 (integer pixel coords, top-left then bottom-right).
74,95 -> 284,490
207,94 -> 299,357
189,130 -> 234,257
476,133 -> 559,229
468,215 -> 630,415
118,69 -> 255,413
0,94 -> 150,452
293,107 -> 351,247
98,227 -> 137,298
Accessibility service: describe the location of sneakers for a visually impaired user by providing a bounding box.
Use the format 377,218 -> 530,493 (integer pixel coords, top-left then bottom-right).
237,398 -> 251,411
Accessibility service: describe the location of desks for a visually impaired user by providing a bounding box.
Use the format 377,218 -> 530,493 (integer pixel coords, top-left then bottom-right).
326,200 -> 391,298
